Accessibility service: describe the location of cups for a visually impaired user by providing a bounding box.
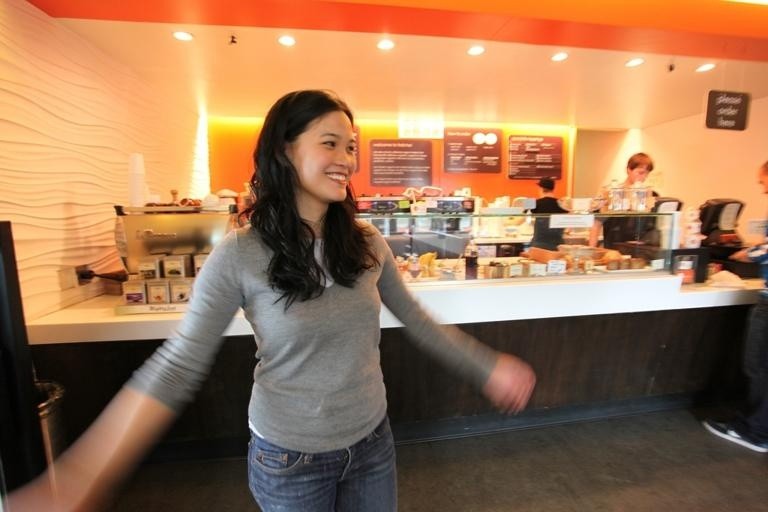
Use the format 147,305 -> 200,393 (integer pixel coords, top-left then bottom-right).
602,179 -> 653,213
130,152 -> 146,207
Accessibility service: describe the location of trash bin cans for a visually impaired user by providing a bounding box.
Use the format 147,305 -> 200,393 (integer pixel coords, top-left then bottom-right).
35,383 -> 64,464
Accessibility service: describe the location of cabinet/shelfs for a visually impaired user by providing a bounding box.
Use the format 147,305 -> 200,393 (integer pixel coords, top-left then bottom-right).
353,211 -> 684,296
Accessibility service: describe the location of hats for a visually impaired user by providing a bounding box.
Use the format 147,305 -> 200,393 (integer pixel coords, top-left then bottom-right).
537,176 -> 555,189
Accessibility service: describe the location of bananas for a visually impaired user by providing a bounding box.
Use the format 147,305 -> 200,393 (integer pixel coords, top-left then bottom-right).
419,251 -> 437,277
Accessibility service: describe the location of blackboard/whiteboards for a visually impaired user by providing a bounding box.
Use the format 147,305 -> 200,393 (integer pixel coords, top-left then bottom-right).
706,90 -> 748,130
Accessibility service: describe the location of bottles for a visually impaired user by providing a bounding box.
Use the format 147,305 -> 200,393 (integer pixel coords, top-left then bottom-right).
465,240 -> 478,280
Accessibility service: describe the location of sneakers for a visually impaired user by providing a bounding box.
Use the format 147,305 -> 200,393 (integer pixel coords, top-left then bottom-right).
703,413 -> 768,453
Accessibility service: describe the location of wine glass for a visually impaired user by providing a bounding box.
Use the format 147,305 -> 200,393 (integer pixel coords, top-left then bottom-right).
408,264 -> 421,283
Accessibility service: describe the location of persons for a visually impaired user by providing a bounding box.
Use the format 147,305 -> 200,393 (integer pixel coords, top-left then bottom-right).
0,89 -> 538,510
514,176 -> 570,252
589,151 -> 655,247
702,161 -> 768,453
